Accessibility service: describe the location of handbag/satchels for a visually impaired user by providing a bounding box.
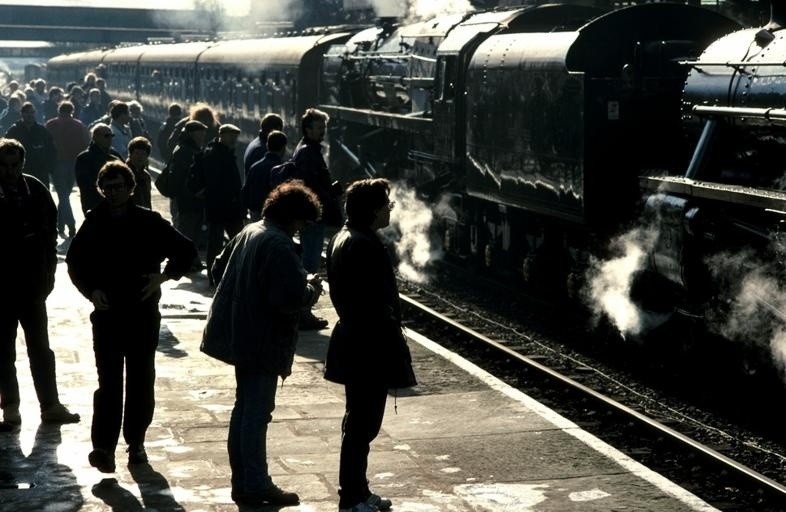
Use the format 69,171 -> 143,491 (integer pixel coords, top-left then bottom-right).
155,167 -> 179,199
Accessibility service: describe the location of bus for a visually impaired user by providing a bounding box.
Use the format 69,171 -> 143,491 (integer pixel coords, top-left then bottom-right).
0,38 -> 55,77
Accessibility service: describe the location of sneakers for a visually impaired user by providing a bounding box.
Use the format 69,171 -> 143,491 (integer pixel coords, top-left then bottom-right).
231,476 -> 298,503
126,443 -> 147,464
41,404 -> 80,425
338,479 -> 392,512
4,403 -> 21,423
88,449 -> 115,473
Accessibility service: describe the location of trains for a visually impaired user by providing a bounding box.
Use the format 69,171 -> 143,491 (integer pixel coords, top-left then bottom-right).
47,0 -> 786,400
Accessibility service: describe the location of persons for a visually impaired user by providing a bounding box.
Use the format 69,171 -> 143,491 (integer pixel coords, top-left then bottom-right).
0,63 -> 416,512
